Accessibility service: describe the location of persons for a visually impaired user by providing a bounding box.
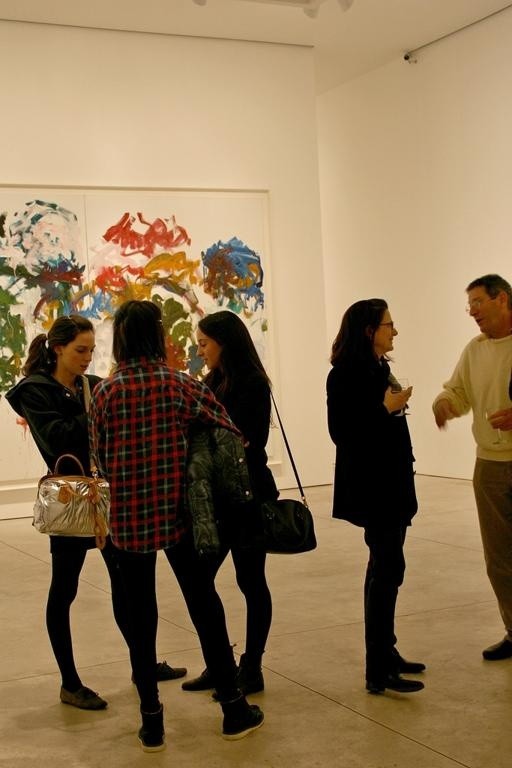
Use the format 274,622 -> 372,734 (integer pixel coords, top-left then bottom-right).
328,293 -> 427,699
432,274 -> 512,658
180,309 -> 274,698
88,298 -> 264,755
21,314 -> 185,708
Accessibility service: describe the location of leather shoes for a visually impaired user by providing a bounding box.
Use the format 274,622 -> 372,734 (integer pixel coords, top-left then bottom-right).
483,640 -> 512,660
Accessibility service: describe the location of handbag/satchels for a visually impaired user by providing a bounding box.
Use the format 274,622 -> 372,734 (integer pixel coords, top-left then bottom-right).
33,454 -> 112,537
262,499 -> 317,553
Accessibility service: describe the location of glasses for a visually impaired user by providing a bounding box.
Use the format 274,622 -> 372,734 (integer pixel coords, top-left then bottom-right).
376,321 -> 392,326
463,299 -> 493,311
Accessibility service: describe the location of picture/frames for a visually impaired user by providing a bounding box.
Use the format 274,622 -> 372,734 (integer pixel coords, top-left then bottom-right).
0,178 -> 287,496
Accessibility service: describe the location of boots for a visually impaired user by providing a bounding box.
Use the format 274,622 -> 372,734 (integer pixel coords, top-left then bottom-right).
366,650 -> 425,695
132,644 -> 264,752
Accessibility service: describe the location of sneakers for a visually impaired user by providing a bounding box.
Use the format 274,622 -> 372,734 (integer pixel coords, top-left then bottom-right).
60,685 -> 107,709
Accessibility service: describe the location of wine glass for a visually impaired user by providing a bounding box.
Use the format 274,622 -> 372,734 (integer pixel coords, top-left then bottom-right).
484,406 -> 509,445
389,378 -> 414,417
